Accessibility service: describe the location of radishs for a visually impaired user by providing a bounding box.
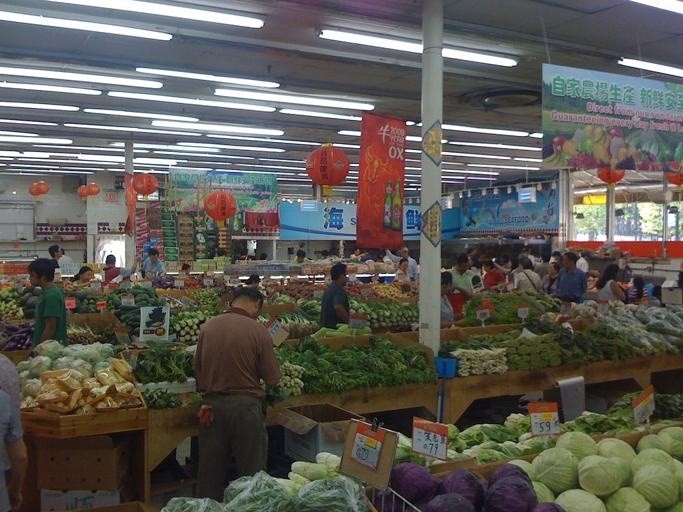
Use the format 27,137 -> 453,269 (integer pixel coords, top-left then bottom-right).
276,451 -> 342,497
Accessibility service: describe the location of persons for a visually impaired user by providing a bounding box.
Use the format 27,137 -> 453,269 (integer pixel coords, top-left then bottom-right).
24,258 -> 67,357
140,249 -> 166,281
0,351 -> 29,512
49,244 -> 63,270
191,284 -> 279,500
100,253 -> 137,280
180,263 -> 192,277
57,248 -> 71,275
68,266 -> 92,287
244,274 -> 267,298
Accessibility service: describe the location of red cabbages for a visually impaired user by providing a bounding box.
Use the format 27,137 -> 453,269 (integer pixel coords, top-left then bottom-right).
373,424 -> 683,512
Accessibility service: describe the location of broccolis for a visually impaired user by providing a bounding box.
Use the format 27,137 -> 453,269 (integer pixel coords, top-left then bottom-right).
504,412 -> 531,432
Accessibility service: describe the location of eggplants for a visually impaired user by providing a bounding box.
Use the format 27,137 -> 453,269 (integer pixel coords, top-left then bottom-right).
114,305 -> 174,337
0,321 -> 35,352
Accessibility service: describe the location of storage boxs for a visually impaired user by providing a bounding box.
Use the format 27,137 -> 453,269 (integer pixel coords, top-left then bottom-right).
134,181 -> 231,273
626,260 -> 683,306
272,403 -> 368,464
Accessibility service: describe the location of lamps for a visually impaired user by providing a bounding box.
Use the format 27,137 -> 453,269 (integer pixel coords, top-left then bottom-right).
395,164 -> 560,205
51,0 -> 267,30
311,24 -> 521,69
